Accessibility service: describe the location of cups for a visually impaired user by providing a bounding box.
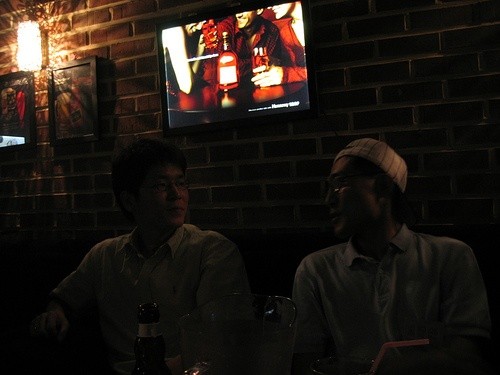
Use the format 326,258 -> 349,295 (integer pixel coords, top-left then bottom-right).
253,47 -> 271,76
310,356 -> 379,375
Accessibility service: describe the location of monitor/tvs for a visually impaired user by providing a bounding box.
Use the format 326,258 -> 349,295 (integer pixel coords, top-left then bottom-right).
155,0 -> 318,139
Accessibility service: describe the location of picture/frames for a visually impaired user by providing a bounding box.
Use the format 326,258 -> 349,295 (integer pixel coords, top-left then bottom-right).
47,55 -> 99,149
0,72 -> 34,153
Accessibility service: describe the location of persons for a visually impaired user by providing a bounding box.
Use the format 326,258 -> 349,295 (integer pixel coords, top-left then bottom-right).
292,138 -> 492,375
29,139 -> 252,375
160,2 -> 308,112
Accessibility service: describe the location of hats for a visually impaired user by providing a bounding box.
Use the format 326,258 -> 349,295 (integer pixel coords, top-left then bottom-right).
332,136 -> 415,213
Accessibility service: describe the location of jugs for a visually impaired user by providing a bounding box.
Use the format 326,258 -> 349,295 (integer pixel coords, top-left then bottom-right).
176,292 -> 298,375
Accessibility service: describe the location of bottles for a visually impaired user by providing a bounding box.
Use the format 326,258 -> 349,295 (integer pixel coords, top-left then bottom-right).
53,83 -> 94,134
133,301 -> 174,375
215,31 -> 240,90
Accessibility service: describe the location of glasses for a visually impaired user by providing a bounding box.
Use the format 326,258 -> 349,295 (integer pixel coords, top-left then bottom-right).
153,178 -> 190,192
